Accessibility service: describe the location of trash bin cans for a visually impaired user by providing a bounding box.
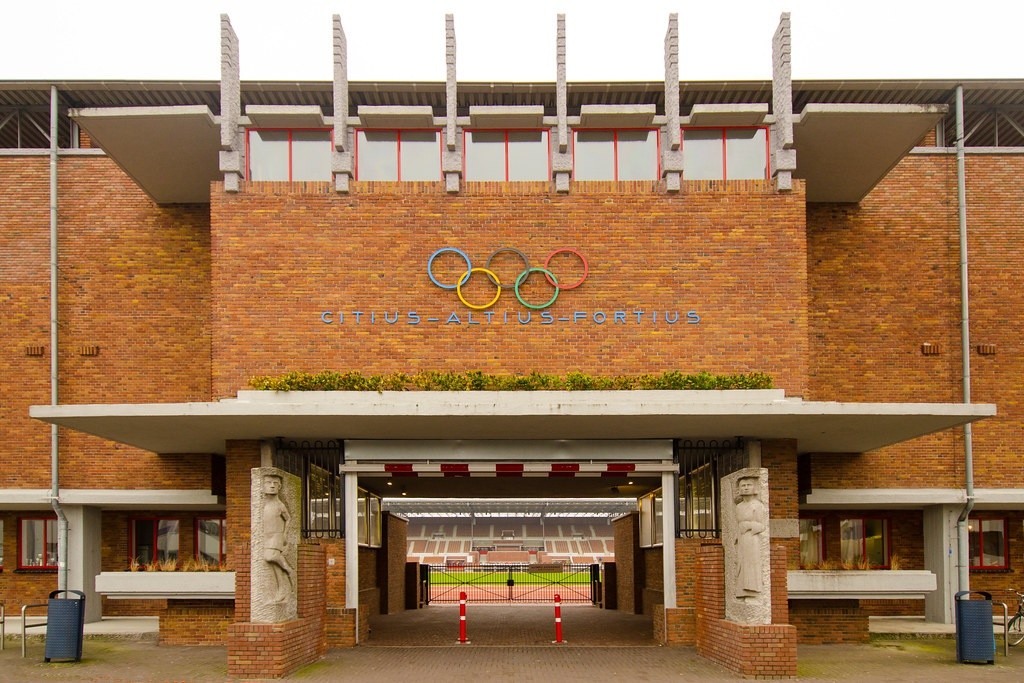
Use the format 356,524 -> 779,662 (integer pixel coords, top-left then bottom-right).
44,590 -> 85,660
954,590 -> 996,664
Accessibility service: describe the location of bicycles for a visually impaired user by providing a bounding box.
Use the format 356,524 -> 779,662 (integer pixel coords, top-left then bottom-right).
1007,589 -> 1024,647
